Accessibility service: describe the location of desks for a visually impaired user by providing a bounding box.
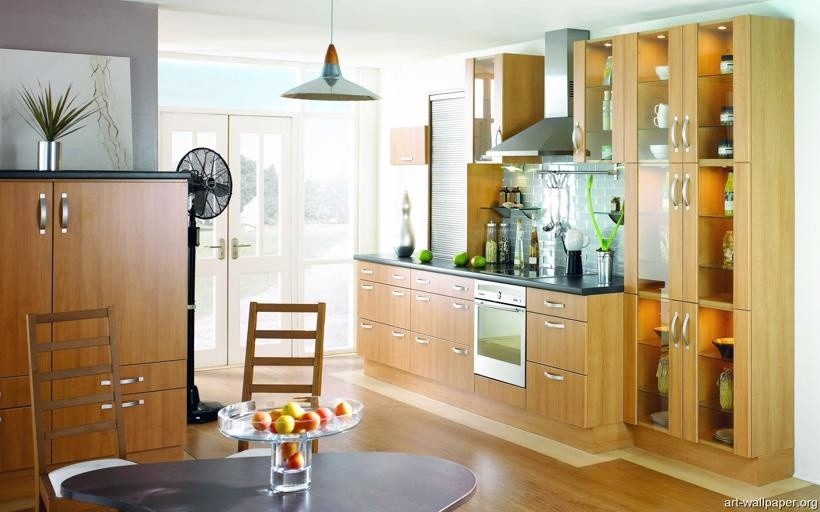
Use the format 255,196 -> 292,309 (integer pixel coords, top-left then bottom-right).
57,448 -> 479,512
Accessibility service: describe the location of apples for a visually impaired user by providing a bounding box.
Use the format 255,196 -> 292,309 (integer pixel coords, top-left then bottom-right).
455,253 -> 485,268
420,250 -> 433,262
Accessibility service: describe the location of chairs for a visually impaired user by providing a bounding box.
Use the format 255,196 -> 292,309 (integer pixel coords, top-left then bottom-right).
22,306 -> 146,512
236,299 -> 328,456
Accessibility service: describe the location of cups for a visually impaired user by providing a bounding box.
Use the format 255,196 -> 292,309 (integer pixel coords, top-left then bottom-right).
652,102 -> 668,128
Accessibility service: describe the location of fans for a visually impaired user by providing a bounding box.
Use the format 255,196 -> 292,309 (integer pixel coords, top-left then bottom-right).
177,146 -> 235,425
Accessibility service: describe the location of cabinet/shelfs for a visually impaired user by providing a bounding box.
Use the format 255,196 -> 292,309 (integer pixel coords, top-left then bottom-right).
412,268 -> 441,397
0,169 -> 191,512
386,125 -> 431,167
379,262 -> 411,389
351,259 -> 379,384
526,285 -> 661,458
624,12 -> 796,489
440,272 -> 477,413
569,34 -> 623,164
463,54 -> 544,164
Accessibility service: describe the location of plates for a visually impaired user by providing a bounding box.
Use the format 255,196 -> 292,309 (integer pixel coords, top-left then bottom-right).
714,426 -> 734,446
647,407 -> 672,426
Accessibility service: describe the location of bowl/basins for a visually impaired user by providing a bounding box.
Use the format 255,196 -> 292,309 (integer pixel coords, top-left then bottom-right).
712,335 -> 737,358
653,326 -> 671,345
649,143 -> 670,160
649,64 -> 674,80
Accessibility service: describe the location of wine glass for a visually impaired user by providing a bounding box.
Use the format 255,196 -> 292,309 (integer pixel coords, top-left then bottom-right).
212,394 -> 368,494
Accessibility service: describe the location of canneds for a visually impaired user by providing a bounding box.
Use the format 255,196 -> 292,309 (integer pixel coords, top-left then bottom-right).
718,139 -> 733,159
719,55 -> 733,74
720,106 -> 733,126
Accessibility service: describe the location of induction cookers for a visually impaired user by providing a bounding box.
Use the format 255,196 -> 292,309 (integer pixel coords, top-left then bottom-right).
473,265 -> 603,304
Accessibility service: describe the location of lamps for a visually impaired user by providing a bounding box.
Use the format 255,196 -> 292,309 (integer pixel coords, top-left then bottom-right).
279,2 -> 382,104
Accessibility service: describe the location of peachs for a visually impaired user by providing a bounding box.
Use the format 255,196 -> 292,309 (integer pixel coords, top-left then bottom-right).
251,400 -> 351,471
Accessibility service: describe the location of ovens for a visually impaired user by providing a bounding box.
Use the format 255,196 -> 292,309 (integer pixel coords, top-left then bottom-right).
472,298 -> 528,390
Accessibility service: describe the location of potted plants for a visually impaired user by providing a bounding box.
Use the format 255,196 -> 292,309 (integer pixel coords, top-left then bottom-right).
12,77 -> 107,171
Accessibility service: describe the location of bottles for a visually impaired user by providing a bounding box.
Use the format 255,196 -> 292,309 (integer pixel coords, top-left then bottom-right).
389,190 -> 417,259
483,219 -> 513,266
600,54 -> 613,85
717,52 -> 735,158
601,90 -> 614,131
600,144 -> 612,160
721,170 -> 734,268
715,367 -> 734,412
566,249 -> 584,275
513,214 -> 540,270
497,185 -> 521,208
655,353 -> 671,395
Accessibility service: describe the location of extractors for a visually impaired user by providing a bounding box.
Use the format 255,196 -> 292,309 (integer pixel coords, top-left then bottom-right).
485,28 -> 591,159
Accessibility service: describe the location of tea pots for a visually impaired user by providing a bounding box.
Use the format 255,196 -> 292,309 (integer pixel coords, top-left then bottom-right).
562,227 -> 590,252
